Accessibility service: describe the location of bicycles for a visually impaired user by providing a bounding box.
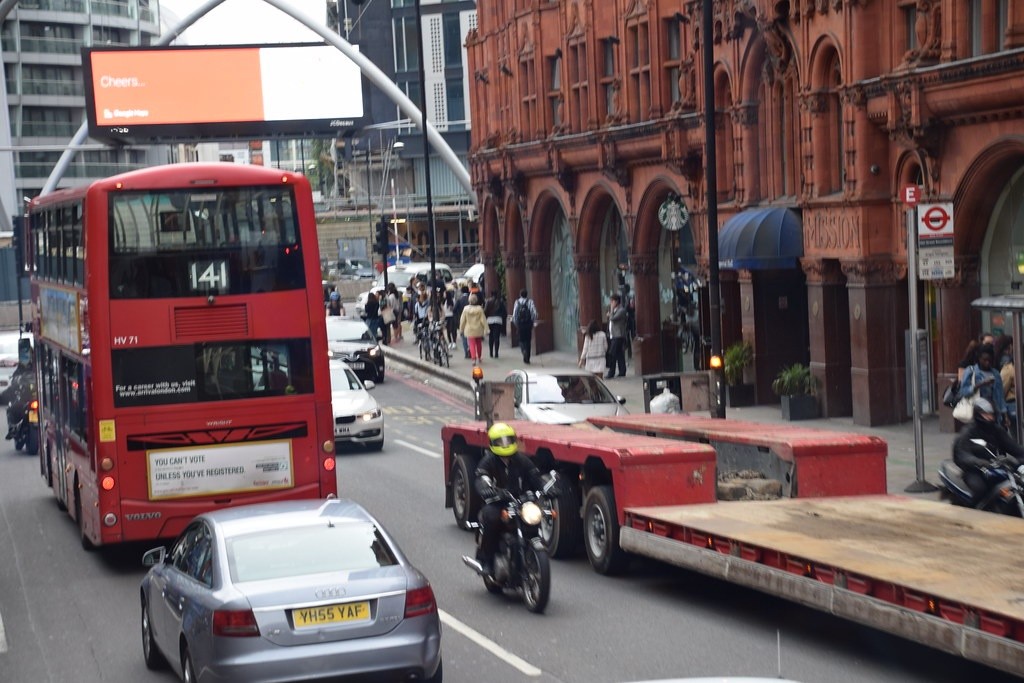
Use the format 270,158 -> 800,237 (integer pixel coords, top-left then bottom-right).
416,320 -> 454,369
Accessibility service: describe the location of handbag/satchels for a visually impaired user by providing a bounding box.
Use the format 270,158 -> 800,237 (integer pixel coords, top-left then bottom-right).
944,385 -> 954,408
953,366 -> 980,423
382,308 -> 396,325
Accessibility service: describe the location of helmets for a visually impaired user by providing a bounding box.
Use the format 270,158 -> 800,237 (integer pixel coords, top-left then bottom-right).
330,292 -> 340,302
488,422 -> 517,456
973,401 -> 994,425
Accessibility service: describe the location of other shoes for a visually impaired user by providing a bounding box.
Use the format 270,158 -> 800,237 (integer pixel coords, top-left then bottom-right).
5,428 -> 16,440
482,561 -> 492,576
604,375 -> 613,378
472,361 -> 477,367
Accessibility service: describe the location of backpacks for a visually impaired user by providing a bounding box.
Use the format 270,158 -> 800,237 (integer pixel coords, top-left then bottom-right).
517,297 -> 531,329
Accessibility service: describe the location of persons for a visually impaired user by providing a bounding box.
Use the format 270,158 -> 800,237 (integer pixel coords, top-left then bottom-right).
453,286 -> 471,357
459,293 -> 490,366
450,280 -> 484,305
5,363 -> 37,440
325,291 -> 345,316
604,294 -> 627,378
952,332 -> 1024,511
578,320 -> 608,380
473,423 -> 560,576
407,271 -> 458,350
365,283 -> 404,345
483,289 -> 507,359
513,289 -> 538,364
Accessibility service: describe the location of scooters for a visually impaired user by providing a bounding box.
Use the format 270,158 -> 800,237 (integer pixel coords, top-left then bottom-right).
938,434 -> 1024,520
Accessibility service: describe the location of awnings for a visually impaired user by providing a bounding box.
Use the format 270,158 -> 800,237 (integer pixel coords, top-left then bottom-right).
717,207 -> 804,270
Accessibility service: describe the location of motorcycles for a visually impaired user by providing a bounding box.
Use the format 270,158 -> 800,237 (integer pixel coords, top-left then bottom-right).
460,468 -> 565,615
8,360 -> 39,457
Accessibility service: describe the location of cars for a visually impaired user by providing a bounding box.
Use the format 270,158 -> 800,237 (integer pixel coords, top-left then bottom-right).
327,358 -> 384,454
502,365 -> 633,426
137,494 -> 446,683
326,311 -> 386,385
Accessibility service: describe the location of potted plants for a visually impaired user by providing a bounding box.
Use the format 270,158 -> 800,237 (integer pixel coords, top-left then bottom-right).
724,341 -> 758,406
773,362 -> 820,421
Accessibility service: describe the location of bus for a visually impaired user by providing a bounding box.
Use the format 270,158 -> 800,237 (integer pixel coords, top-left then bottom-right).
8,154 -> 340,556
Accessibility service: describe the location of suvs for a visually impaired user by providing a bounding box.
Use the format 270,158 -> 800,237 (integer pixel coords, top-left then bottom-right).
355,259 -> 454,325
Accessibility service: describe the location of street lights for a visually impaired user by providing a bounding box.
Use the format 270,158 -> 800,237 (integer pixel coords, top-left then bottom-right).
392,140 -> 405,218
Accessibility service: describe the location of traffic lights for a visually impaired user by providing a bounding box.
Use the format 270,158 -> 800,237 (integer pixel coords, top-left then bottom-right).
375,221 -> 389,255
